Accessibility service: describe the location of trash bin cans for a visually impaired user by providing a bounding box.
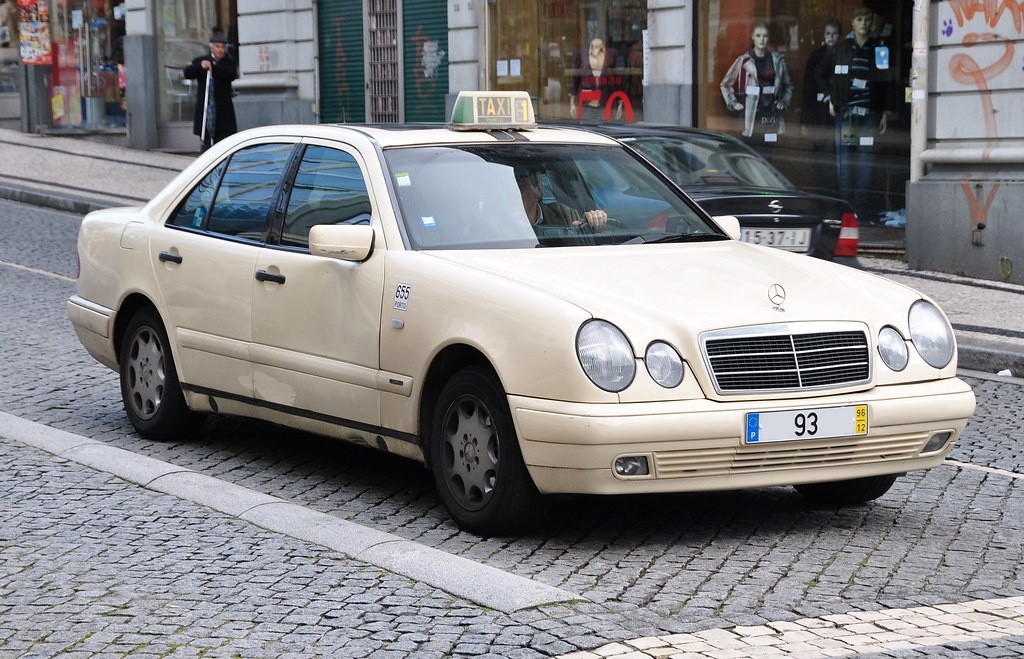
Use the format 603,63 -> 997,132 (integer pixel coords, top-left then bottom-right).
232,74 -> 299,132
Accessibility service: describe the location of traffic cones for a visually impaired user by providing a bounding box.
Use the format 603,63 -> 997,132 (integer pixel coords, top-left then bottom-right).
832,213 -> 864,270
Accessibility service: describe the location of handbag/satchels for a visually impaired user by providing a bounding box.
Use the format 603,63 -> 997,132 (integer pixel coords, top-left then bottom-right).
18,11 -> 52,65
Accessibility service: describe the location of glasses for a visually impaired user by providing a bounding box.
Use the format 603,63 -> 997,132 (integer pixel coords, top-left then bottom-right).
510,183 -> 534,193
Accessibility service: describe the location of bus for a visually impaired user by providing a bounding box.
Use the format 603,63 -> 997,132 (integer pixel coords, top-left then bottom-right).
534,118 -> 862,270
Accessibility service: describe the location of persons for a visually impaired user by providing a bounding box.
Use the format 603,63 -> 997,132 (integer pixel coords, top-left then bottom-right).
542,40 -> 563,119
183,34 -> 240,157
513,165 -> 609,232
719,24 -> 793,170
568,37 -> 626,124
799,5 -> 899,226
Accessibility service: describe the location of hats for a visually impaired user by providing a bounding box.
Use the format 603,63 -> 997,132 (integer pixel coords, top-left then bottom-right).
210,26 -> 228,43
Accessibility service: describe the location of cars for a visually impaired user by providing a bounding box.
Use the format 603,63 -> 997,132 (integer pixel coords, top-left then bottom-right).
64,91 -> 976,537
